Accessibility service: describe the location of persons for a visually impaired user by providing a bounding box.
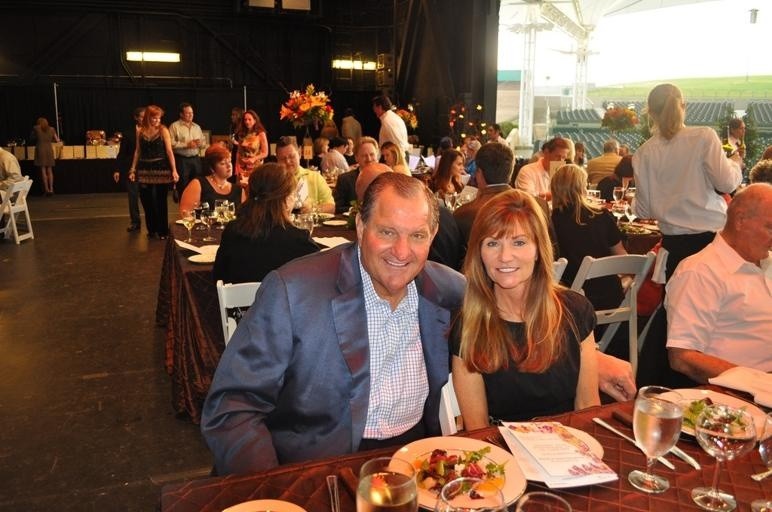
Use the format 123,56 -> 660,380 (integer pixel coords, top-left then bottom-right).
0,146 -> 25,240
452,143 -> 515,251
428,124 -> 509,206
515,137 -> 634,310
311,134 -> 469,272
228,108 -> 268,185
275,136 -> 335,213
30,117 -> 62,197
126,104 -> 181,242
212,165 -> 320,325
369,94 -> 411,163
660,180 -> 771,393
111,106 -> 151,233
749,159 -> 772,185
180,143 -> 247,220
762,145 -> 772,160
722,117 -> 746,174
445,188 -> 602,433
198,170 -> 639,479
630,83 -> 748,391
168,102 -> 208,202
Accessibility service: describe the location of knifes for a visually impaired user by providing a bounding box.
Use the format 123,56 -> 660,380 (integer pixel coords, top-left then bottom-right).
590,415 -> 676,470
613,406 -> 700,470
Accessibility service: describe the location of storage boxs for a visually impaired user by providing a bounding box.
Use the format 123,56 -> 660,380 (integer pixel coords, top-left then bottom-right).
377,70 -> 395,87
376,53 -> 395,69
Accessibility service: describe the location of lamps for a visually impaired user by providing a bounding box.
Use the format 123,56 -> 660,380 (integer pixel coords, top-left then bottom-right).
508,19 -> 558,40
124,48 -> 182,64
331,57 -> 378,71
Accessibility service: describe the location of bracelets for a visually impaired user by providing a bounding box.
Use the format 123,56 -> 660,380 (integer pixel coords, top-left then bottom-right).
128,168 -> 137,173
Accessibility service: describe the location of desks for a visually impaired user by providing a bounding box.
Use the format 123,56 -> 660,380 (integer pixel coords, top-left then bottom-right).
1,141 -> 132,196
162,116 -> 771,246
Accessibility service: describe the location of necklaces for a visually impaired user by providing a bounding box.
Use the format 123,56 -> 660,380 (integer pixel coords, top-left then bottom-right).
210,176 -> 227,189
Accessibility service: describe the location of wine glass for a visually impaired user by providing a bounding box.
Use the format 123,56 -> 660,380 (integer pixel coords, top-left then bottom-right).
313,200 -> 322,226
691,401 -> 758,512
751,413 -> 772,512
184,199 -> 235,244
626,386 -> 684,497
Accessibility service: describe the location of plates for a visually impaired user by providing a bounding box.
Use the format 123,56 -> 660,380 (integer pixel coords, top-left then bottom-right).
563,422 -> 603,461
390,436 -> 530,510
318,212 -> 335,219
323,220 -> 348,226
625,228 -> 651,235
225,498 -> 308,511
652,388 -> 771,441
176,217 -> 202,225
187,253 -> 215,265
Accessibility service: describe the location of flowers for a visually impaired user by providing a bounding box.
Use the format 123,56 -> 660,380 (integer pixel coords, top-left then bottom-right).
278,82 -> 341,129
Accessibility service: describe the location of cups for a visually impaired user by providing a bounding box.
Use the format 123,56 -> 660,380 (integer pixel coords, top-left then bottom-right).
297,213 -> 315,237
516,490 -> 572,511
585,186 -> 636,226
442,191 -> 474,210
433,478 -> 508,511
355,457 -> 418,512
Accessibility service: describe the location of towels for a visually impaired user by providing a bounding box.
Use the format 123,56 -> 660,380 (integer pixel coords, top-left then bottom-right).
706,365 -> 772,409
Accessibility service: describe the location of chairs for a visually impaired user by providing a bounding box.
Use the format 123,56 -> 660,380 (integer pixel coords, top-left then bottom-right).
565,249 -> 659,379
549,255 -> 570,286
555,94 -> 767,163
213,279 -> 272,351
2,175 -> 35,244
